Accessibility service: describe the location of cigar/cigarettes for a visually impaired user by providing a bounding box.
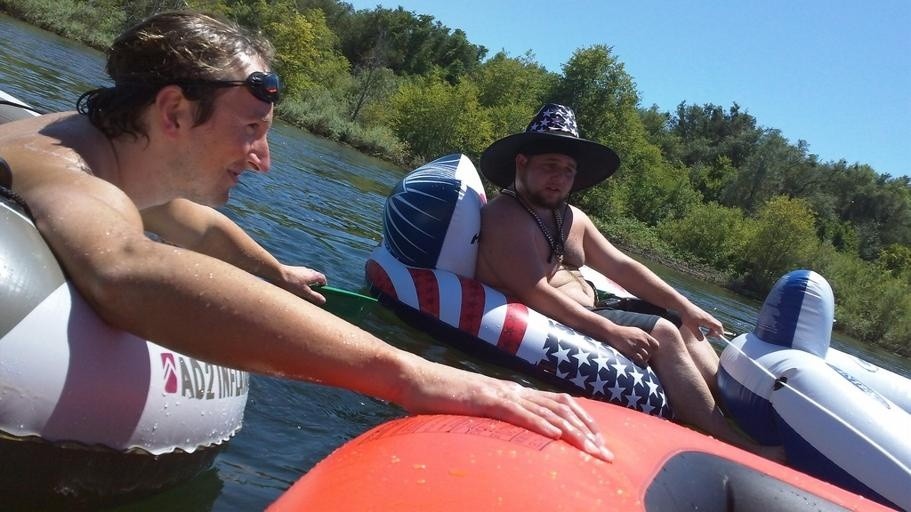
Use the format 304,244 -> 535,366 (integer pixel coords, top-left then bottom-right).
724,331 -> 736,336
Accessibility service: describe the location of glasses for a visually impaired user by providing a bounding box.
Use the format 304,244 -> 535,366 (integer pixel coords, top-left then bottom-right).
185,71 -> 280,104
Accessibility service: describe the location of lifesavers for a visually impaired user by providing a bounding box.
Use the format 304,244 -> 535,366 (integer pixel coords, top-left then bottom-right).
0,89 -> 251,459
263,397 -> 896,511
715,271 -> 911,510
365,154 -> 679,422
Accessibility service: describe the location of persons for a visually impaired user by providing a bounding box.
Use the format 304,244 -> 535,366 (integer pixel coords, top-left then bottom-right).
0,10 -> 613,464
474,103 -> 785,466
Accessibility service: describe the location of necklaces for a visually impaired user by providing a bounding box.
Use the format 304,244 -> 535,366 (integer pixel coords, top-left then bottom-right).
501,184 -> 565,264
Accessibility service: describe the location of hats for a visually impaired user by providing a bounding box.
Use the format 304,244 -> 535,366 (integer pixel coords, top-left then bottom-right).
480,102 -> 620,193
